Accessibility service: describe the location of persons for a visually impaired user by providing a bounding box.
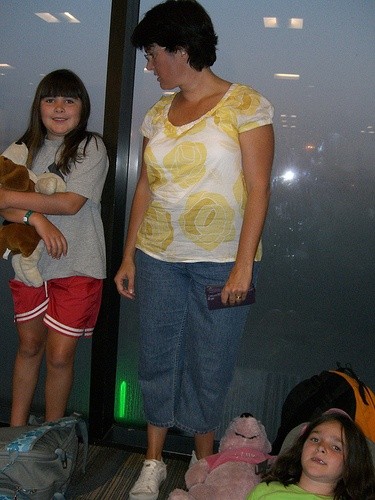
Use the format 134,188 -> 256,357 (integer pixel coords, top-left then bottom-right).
113,0 -> 277,500
240,410 -> 375,500
0,69 -> 110,429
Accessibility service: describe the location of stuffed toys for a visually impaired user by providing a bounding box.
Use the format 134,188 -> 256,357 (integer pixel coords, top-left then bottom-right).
168,409 -> 274,500
0,139 -> 68,288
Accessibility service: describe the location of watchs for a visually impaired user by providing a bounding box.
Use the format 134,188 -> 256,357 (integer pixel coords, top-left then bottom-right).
23,209 -> 34,225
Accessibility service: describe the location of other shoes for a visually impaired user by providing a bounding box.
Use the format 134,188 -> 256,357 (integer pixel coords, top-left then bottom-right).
129,458 -> 167,500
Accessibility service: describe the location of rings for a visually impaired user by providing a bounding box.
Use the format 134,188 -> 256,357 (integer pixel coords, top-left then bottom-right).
236,296 -> 241,299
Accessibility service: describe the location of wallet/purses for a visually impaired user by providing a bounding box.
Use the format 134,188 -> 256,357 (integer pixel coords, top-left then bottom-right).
205,283 -> 256,310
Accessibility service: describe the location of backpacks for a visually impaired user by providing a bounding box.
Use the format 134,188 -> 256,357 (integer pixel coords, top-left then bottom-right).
0,412 -> 88,500
268,362 -> 375,456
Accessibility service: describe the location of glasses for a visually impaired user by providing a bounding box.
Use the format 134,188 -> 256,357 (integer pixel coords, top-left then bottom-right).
144,47 -> 166,61
